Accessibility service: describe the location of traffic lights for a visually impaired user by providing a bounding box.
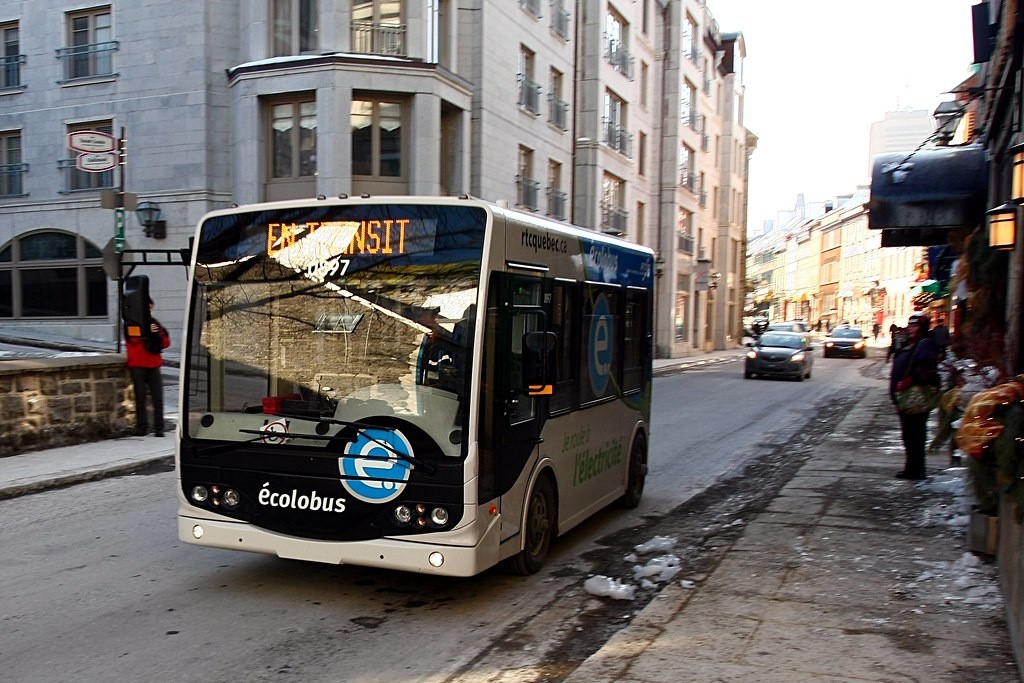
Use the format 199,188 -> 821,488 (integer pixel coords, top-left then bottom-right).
113,208 -> 125,252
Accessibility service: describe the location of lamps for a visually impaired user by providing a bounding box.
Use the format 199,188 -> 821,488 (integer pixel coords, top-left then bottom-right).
710,271 -> 722,290
1007,131 -> 1024,204
135,201 -> 167,240
655,257 -> 667,280
986,201 -> 1018,251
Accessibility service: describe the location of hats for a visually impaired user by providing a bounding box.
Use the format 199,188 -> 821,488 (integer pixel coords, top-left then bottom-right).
908,314 -> 929,335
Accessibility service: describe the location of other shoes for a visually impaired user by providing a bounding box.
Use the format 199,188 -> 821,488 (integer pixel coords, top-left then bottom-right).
894,470 -> 926,481
155,429 -> 164,437
131,428 -> 147,435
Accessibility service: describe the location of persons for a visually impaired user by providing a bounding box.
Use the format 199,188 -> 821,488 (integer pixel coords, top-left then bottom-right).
889,324 -> 897,342
873,324 -> 879,339
932,319 -> 951,358
415,310 -> 465,368
890,313 -> 935,481
123,299 -> 170,437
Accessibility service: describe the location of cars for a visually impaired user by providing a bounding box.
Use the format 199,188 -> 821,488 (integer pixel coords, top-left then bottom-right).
824,327 -> 869,357
743,332 -> 814,381
747,318 -> 808,333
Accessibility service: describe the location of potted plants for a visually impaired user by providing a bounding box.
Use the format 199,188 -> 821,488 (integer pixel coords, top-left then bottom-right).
964,448 -> 1000,557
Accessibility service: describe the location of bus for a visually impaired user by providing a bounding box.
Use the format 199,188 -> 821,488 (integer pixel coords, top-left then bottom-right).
172,194 -> 659,581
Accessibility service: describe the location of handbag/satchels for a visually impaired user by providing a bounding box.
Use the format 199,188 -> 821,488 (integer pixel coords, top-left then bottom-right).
894,383 -> 940,417
